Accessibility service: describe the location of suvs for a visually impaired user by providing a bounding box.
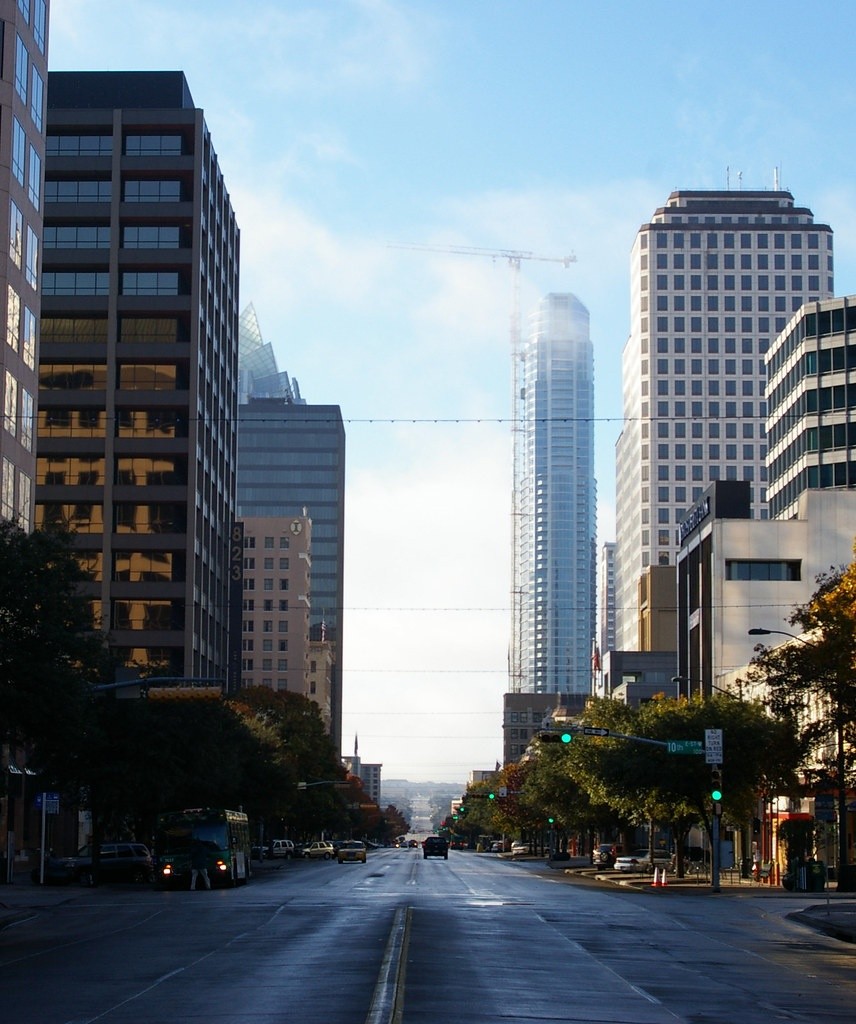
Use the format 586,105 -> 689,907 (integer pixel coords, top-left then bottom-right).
33,842 -> 156,889
421,837 -> 450,860
590,844 -> 643,870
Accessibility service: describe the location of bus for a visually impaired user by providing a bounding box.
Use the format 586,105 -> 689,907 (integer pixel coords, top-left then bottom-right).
155,808 -> 253,889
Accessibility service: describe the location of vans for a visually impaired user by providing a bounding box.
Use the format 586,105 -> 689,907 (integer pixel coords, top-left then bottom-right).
271,839 -> 295,860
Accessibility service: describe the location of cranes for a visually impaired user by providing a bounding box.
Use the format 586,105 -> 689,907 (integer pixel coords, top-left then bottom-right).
383,237 -> 580,344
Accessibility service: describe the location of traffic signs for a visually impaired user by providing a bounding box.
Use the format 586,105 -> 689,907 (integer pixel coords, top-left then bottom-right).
583,726 -> 610,737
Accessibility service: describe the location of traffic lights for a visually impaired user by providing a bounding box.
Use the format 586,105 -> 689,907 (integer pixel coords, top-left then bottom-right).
710,769 -> 723,802
541,732 -> 573,743
548,813 -> 556,828
469,793 -> 496,798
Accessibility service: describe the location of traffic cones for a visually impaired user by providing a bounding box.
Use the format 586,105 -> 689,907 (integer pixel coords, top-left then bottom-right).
660,869 -> 667,886
650,866 -> 661,887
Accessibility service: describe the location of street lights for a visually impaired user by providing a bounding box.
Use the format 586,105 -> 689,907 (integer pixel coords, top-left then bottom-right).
747,627 -> 852,893
671,675 -> 751,878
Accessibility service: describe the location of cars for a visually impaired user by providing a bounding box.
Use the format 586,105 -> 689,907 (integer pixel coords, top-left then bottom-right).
493,841 -> 510,852
337,841 -> 367,864
512,842 -> 550,854
451,837 -> 464,850
409,840 -> 418,847
332,840 -> 346,860
251,846 -> 270,859
613,849 -> 674,873
294,841 -> 335,860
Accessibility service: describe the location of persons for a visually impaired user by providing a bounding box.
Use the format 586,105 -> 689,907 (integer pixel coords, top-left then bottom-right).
190,841 -> 211,891
332,839 -> 338,859
808,854 -> 815,861
672,851 -> 691,874
